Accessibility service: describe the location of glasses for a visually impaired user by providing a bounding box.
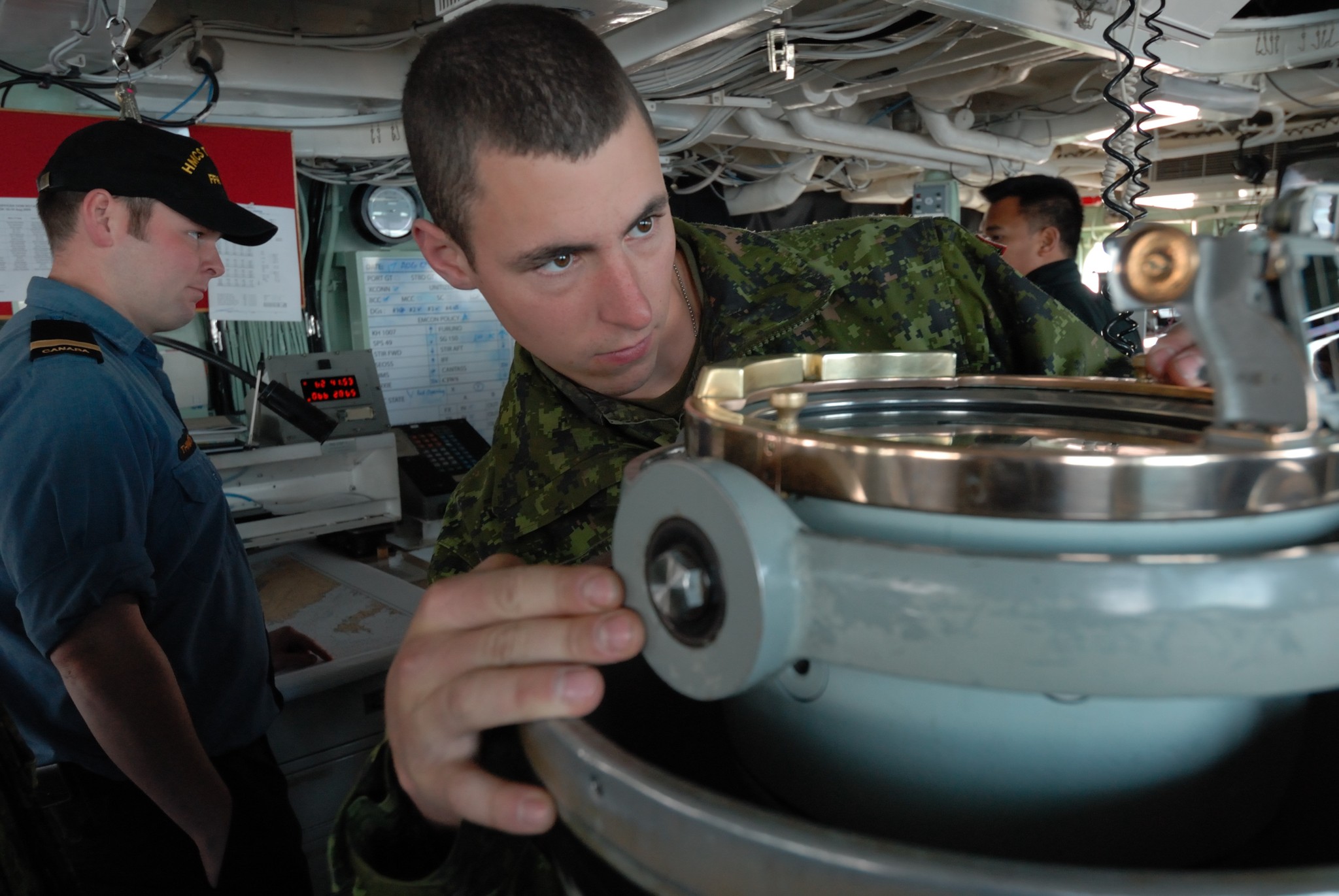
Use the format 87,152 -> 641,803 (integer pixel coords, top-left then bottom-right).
977,226 -> 1050,244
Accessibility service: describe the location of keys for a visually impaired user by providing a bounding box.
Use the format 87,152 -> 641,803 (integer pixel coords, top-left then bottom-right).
105,15 -> 143,127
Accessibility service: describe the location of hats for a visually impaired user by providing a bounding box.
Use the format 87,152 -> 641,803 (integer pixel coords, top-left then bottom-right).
35,117 -> 278,246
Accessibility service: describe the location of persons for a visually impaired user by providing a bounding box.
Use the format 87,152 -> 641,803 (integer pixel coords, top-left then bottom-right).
1,121 -> 321,896
321,0 -> 1211,896
968,175 -> 1145,372
